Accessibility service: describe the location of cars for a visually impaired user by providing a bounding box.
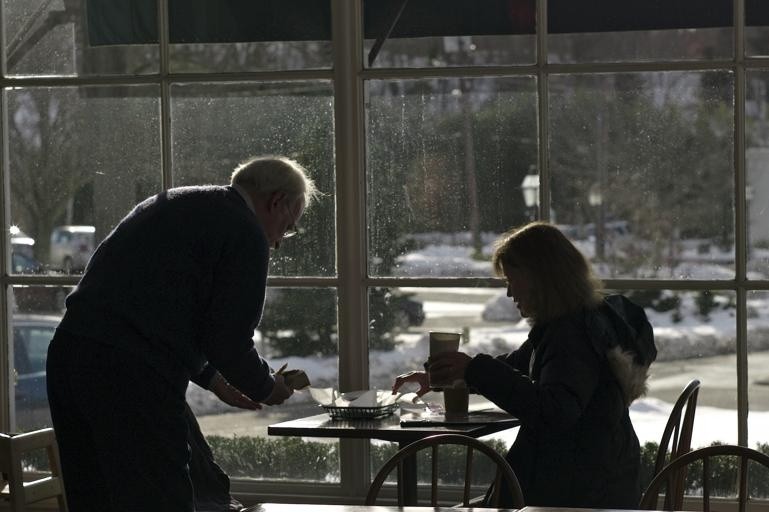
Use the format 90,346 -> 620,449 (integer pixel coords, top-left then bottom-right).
7,236 -> 37,275
46,225 -> 93,275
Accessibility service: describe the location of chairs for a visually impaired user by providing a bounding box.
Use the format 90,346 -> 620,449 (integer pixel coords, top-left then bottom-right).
364,433 -> 527,510
635,442 -> 768,510
650,378 -> 701,511
0,427 -> 70,511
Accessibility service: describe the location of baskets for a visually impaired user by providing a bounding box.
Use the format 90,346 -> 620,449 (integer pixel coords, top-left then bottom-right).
319,403 -> 398,418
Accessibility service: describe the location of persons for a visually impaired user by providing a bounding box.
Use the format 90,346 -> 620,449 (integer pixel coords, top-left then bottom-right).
45,157 -> 318,512
391,221 -> 658,508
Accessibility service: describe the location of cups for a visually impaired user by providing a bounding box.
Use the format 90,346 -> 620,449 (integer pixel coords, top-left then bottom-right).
429,332 -> 468,418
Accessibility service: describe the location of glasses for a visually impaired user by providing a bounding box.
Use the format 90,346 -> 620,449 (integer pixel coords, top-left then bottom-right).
284,203 -> 299,239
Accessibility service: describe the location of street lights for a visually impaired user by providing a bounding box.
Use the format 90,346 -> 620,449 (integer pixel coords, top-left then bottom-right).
588,181 -> 606,262
521,174 -> 544,225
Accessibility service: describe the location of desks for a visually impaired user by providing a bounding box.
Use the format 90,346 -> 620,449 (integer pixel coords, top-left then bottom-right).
265,405 -> 523,507
